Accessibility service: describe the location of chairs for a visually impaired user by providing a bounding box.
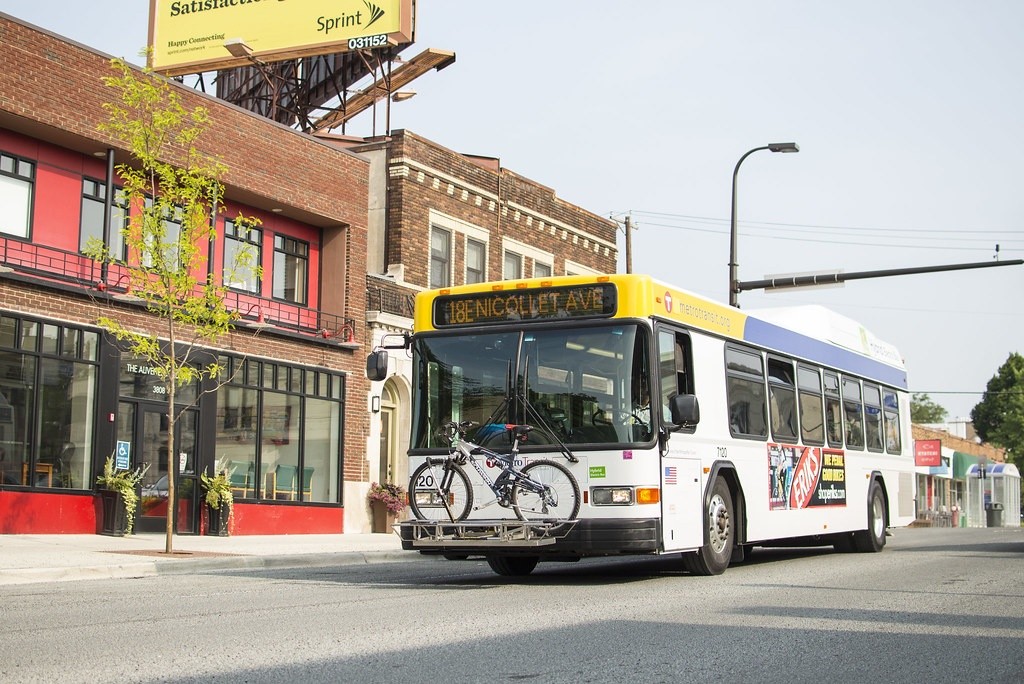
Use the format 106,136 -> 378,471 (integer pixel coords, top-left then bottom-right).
225,461 -> 315,501
34,441 -> 75,488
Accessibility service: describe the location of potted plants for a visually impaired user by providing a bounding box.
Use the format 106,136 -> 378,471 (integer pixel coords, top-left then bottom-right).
96,450 -> 152,537
200,454 -> 235,538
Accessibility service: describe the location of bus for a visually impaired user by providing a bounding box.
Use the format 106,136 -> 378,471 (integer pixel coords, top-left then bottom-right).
367,275 -> 916,581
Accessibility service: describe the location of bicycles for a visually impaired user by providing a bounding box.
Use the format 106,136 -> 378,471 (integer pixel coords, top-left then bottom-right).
407,420 -> 581,536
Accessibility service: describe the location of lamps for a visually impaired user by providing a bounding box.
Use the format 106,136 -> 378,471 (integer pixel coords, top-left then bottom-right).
99,276 -> 143,301
322,324 -> 363,348
372,395 -> 381,414
238,304 -> 274,328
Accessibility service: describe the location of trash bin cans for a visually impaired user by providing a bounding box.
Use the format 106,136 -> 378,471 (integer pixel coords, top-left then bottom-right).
985,502 -> 1005,528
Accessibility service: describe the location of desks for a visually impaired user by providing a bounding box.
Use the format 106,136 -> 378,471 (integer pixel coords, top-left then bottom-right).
0,462 -> 54,487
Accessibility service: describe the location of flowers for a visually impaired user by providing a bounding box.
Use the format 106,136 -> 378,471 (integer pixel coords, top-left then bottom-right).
366,481 -> 406,518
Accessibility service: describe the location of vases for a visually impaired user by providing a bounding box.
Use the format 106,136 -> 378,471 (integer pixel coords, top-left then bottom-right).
373,502 -> 394,533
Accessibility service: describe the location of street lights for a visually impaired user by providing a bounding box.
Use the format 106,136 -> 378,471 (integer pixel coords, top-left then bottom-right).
729,142 -> 802,311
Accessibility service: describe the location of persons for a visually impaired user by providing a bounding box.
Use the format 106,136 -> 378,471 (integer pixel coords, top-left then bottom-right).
618,373 -> 672,424
